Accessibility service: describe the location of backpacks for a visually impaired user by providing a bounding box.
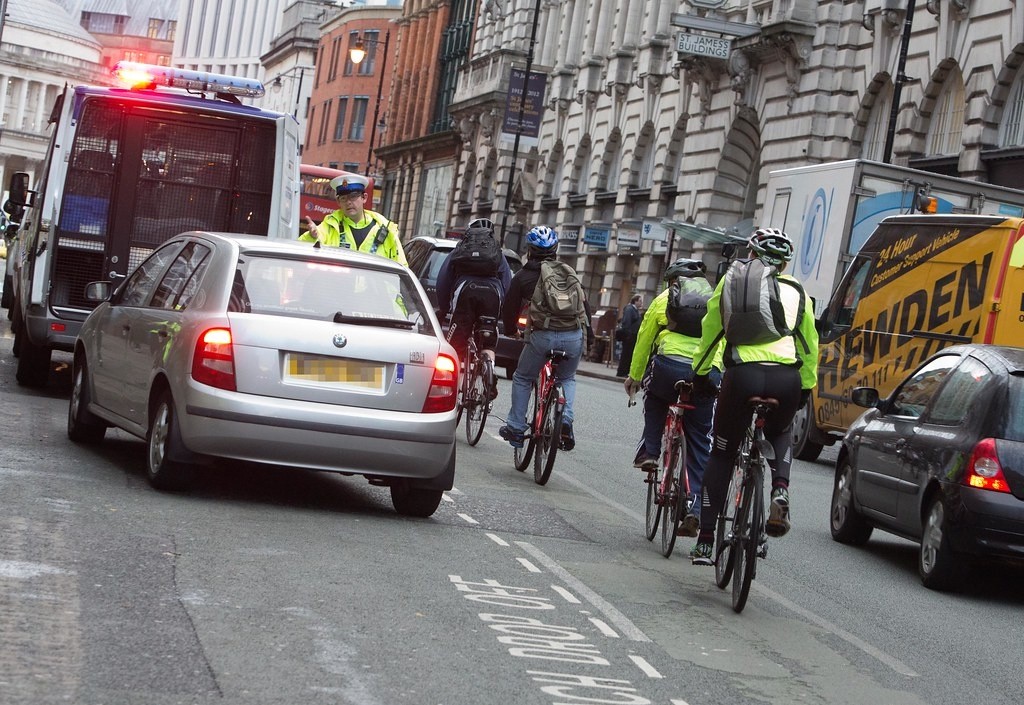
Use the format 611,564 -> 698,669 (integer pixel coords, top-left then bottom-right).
720,258 -> 811,346
448,233 -> 505,294
522,260 -> 584,332
654,276 -> 714,346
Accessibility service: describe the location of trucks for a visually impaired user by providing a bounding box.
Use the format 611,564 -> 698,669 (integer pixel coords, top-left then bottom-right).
717,156 -> 1024,334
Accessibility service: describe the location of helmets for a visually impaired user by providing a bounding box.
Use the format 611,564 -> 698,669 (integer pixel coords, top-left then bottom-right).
746,228 -> 795,265
467,218 -> 495,235
663,259 -> 707,281
526,226 -> 558,259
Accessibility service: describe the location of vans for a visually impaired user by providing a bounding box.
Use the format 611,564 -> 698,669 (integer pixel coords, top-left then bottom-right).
787,211 -> 1024,460
1,60 -> 302,386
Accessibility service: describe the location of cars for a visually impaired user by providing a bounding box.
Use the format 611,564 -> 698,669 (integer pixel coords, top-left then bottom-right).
824,342 -> 1024,598
66,230 -> 465,520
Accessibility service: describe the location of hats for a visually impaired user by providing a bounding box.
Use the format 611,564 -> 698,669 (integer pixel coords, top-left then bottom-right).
329,174 -> 369,195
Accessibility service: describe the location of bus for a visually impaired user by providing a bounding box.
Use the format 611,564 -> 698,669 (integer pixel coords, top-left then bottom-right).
298,162 -> 376,241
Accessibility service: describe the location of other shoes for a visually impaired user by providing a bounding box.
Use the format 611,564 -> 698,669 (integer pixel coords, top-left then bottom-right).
687,542 -> 713,566
633,448 -> 659,469
559,417 -> 575,451
498,426 -> 524,449
678,511 -> 700,538
765,487 -> 790,537
483,371 -> 498,402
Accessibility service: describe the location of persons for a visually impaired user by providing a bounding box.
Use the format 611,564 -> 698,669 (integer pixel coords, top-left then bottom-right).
438,218 -> 512,402
616,295 -> 643,377
689,228 -> 819,559
297,175 -> 409,320
625,257 -> 727,537
499,226 -> 595,451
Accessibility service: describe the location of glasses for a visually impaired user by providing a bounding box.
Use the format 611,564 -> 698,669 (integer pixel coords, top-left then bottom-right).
337,192 -> 363,202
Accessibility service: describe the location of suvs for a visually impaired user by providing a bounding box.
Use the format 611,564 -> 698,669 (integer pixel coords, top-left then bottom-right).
397,223 -> 537,381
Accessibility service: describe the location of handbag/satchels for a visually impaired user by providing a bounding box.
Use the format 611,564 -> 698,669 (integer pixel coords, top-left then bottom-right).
614,323 -> 624,342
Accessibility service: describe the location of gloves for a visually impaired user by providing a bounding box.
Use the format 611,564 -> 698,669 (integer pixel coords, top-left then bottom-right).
797,389 -> 812,410
693,373 -> 720,398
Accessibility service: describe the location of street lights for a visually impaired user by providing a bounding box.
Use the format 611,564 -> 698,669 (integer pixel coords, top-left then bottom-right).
346,26 -> 392,176
271,66 -> 305,119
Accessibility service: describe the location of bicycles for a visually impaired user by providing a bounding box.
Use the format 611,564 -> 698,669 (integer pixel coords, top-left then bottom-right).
627,359 -> 702,560
705,396 -> 783,615
508,333 -> 598,487
454,307 -> 503,446
